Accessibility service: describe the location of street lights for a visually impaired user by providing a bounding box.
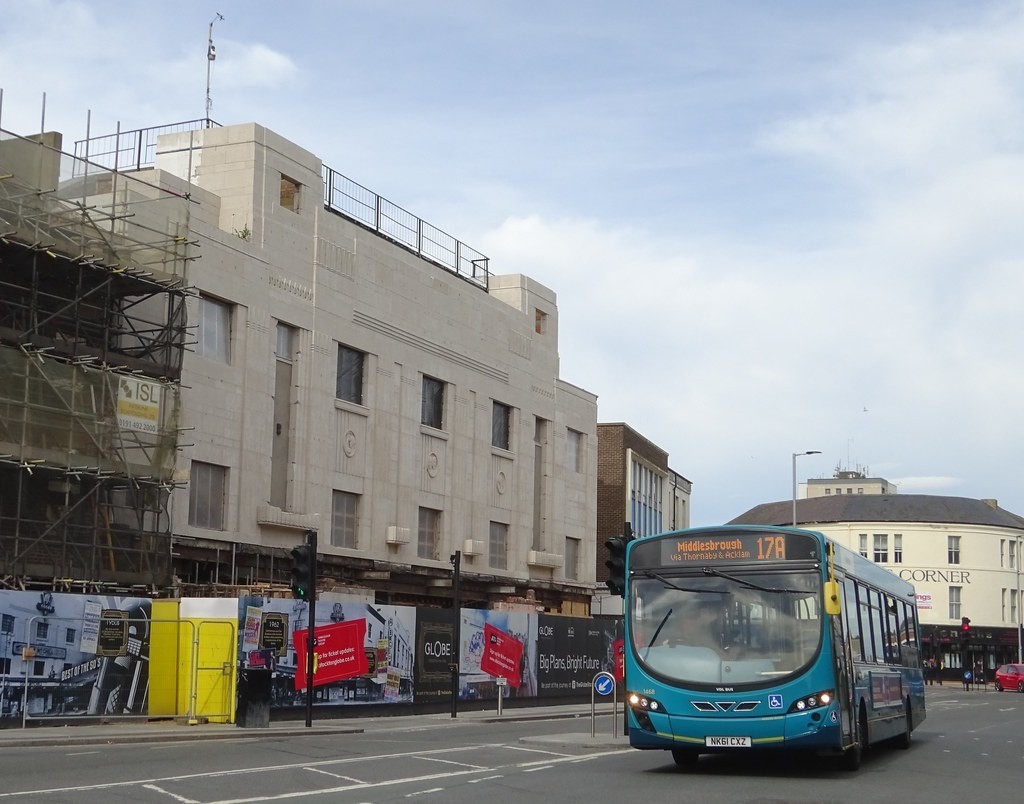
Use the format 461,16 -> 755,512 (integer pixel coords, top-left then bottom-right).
792,449 -> 824,527
1015,534 -> 1024,663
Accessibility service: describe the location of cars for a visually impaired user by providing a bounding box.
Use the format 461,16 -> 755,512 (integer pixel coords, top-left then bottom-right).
994,664 -> 1024,693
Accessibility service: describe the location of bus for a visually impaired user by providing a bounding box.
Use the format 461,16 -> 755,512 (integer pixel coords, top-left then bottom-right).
622,522 -> 926,769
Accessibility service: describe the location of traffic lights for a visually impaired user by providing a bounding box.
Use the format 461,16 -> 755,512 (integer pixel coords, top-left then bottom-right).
291,546 -> 314,602
604,536 -> 626,595
960,615 -> 970,643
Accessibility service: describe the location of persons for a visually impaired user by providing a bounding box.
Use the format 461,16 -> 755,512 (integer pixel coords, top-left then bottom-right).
514,632 -> 529,668
924,663 -> 942,685
469,631 -> 485,654
662,598 -> 741,660
49,665 -> 56,679
